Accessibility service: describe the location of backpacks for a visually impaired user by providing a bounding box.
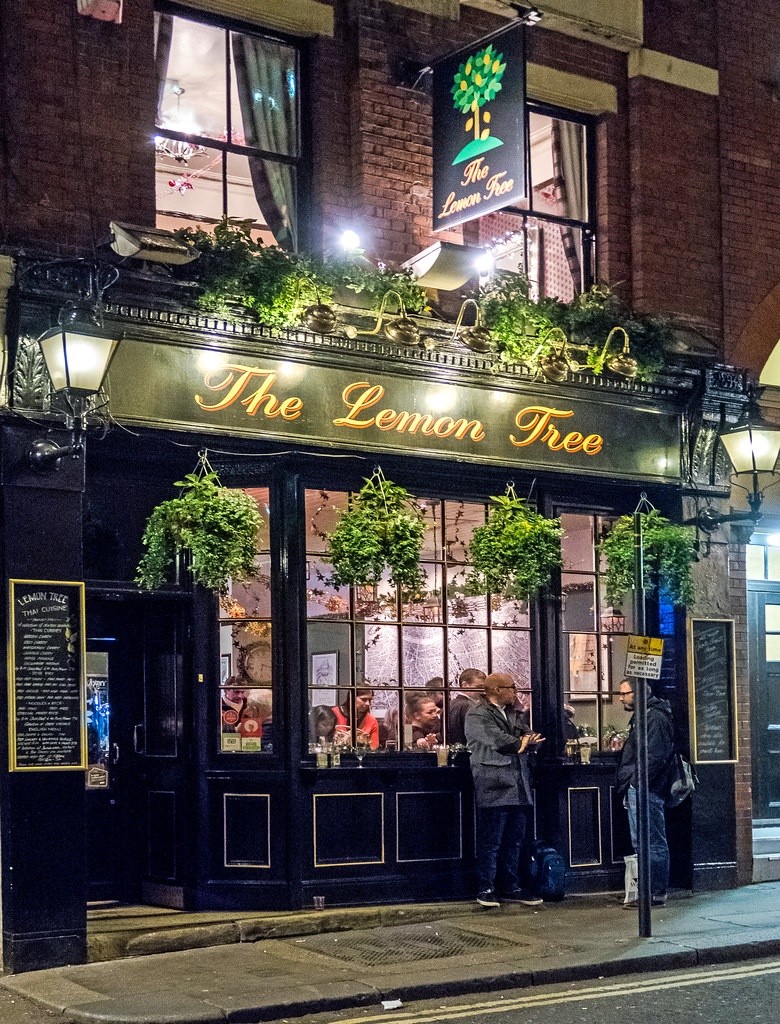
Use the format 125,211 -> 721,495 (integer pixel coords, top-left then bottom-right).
528,841 -> 567,902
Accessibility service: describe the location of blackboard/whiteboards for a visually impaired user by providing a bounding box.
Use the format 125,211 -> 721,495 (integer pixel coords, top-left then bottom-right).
688,617 -> 741,763
7,578 -> 90,772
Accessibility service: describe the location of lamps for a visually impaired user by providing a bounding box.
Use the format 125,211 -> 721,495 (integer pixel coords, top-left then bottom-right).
424,298 -> 495,355
697,384 -> 780,533
290,276 -> 337,334
446,541 -> 460,568
401,240 -> 488,291
157,86 -> 210,166
345,288 -> 422,348
25,287 -> 125,476
600,606 -> 625,632
501,325 -> 570,385
423,564 -> 440,623
355,580 -> 380,603
110,221 -> 203,267
658,320 -> 720,359
570,327 -> 639,378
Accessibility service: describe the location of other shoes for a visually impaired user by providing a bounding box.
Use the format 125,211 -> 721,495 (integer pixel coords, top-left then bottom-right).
501,890 -> 542,906
622,898 -> 665,911
476,892 -> 501,907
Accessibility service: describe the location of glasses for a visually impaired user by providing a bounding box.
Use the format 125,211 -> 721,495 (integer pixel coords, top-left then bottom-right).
620,690 -> 634,697
492,683 -> 517,690
358,697 -> 374,704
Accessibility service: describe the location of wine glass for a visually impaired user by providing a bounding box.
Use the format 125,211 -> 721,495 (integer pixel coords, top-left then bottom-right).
450,744 -> 459,768
354,738 -> 367,769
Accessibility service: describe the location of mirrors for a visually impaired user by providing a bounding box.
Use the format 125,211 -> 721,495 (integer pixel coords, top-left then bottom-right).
489,223 -> 541,301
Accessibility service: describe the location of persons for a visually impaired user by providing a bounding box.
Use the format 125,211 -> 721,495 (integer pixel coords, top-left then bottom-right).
618,677 -> 676,909
309,668 -> 578,751
221,676 -> 273,750
464,671 -> 546,906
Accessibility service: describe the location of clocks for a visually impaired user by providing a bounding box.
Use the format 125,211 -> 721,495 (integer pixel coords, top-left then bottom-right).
238,640 -> 271,686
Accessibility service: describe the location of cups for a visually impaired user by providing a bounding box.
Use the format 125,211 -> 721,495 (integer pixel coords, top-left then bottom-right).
313,896 -> 326,911
308,742 -> 332,754
580,744 -> 590,765
436,742 -> 449,767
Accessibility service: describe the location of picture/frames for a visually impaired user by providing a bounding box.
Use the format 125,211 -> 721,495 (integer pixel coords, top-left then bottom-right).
310,650 -> 339,708
221,652 -> 232,685
568,629 -> 614,704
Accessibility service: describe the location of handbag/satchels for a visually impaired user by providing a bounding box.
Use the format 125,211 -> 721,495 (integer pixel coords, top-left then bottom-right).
669,749 -> 699,809
624,854 -> 639,904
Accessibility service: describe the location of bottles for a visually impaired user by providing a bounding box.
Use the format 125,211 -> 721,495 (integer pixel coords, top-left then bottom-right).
317,735 -> 328,767
331,736 -> 340,768
